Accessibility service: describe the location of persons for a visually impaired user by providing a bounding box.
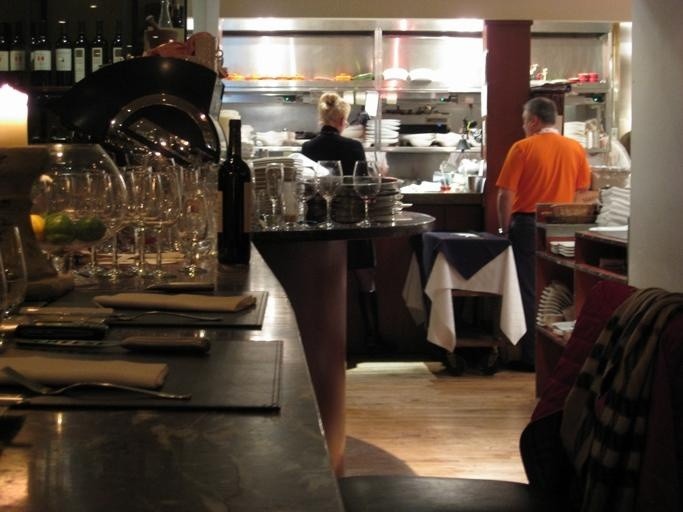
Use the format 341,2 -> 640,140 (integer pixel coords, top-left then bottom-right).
301,91 -> 368,178
495,97 -> 592,272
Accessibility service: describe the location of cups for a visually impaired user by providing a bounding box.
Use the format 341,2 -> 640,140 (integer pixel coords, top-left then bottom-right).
468,176 -> 486,193
440,173 -> 452,189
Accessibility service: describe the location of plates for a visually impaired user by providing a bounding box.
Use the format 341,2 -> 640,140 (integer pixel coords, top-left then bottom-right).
365,118 -> 402,147
551,241 -> 576,259
252,157 -> 295,215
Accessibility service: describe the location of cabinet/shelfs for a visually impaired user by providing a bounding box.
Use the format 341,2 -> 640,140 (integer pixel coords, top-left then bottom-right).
216,16 -> 622,207
422,229 -> 512,375
1,1 -> 189,225
533,201 -> 628,399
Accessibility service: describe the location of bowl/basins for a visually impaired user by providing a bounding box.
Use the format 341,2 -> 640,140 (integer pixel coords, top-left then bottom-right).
405,133 -> 435,146
436,133 -> 461,146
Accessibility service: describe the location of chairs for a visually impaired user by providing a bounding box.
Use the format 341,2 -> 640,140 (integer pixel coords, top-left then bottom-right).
337,282 -> 683,512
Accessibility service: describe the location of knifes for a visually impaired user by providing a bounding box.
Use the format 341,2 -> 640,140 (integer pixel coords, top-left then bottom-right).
12,336 -> 211,352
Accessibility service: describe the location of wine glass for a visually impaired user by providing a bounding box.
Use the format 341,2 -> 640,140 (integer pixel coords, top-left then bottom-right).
0,225 -> 28,351
266,161 -> 382,231
51,146 -> 213,278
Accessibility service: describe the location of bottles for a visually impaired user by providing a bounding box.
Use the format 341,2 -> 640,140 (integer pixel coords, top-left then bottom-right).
0,0 -> 189,93
216,120 -> 251,266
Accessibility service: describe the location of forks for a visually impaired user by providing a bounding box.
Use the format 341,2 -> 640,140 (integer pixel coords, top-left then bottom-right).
94,300 -> 224,322
2,365 -> 192,401
